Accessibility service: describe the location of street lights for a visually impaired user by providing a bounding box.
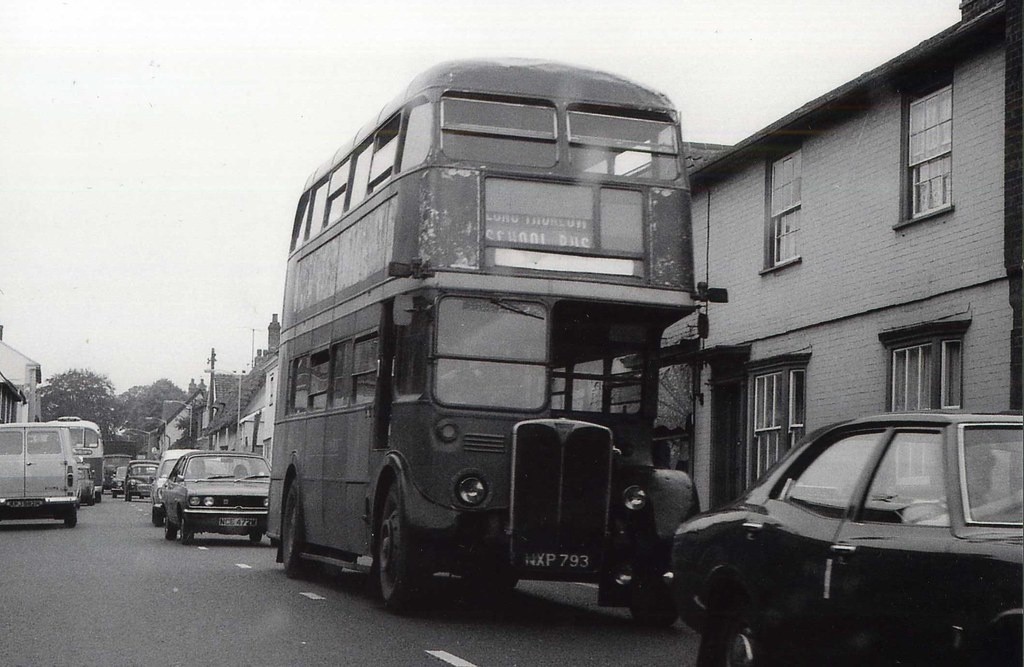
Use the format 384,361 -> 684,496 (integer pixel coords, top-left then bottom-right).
145,416 -> 167,449
163,400 -> 192,448
133,429 -> 151,454
204,368 -> 242,452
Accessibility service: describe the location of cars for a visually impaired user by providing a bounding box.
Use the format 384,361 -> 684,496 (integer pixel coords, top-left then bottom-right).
151,449 -> 217,528
162,450 -> 272,543
102,454 -> 161,501
671,408 -> 1024,667
75,455 -> 97,507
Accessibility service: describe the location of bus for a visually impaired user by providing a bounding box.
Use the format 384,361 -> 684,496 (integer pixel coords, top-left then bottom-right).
266,55 -> 705,621
48,416 -> 104,507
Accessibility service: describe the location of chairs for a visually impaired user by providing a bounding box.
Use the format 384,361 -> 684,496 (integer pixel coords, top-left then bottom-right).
188,458 -> 205,477
233,464 -> 248,477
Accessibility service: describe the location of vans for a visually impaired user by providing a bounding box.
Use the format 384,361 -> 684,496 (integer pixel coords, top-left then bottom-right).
0,422 -> 84,529
